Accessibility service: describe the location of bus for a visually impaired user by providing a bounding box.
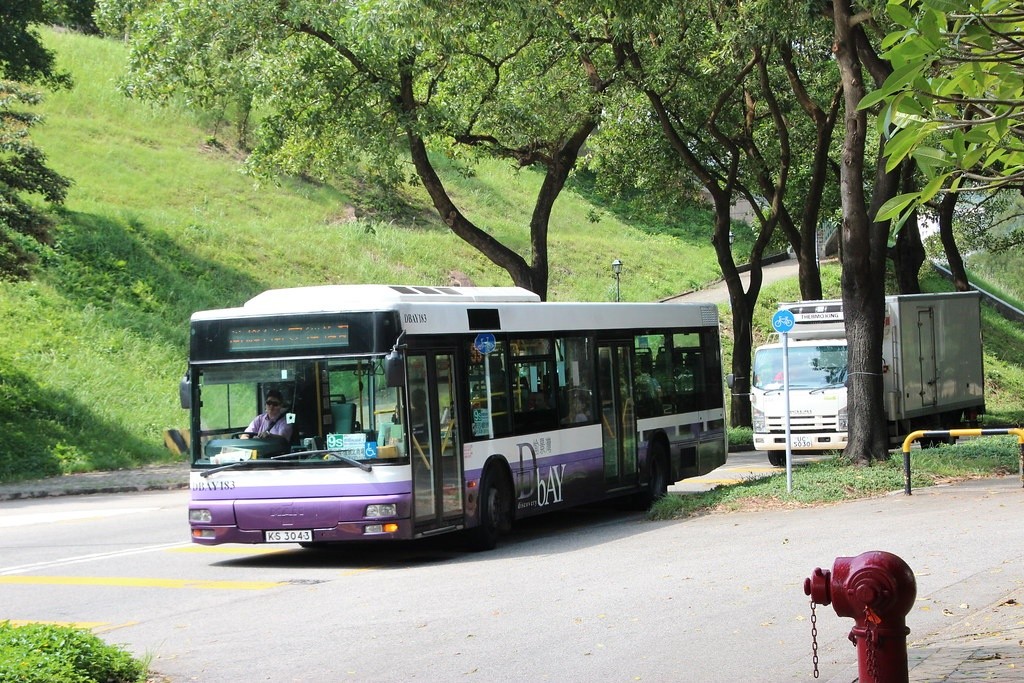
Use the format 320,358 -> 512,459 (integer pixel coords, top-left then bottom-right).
179,284 -> 729,554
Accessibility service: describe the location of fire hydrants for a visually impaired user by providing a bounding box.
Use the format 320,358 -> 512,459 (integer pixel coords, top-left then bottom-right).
803,551 -> 917,683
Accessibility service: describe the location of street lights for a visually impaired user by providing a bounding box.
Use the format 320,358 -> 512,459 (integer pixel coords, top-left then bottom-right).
612,260 -> 624,302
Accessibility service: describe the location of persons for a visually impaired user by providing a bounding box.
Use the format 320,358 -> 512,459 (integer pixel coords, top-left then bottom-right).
392,388 -> 430,446
519,388 -> 558,432
240,390 -> 292,447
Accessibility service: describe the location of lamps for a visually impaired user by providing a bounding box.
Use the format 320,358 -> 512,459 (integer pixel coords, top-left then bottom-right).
729,231 -> 735,243
611,260 -> 623,273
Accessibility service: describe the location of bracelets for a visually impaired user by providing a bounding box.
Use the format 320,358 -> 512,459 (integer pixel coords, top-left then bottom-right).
266,431 -> 270,435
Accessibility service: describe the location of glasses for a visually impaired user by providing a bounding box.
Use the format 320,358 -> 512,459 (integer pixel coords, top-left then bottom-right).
266,400 -> 283,406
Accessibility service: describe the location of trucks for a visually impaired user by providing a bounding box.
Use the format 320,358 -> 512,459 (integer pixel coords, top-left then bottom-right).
728,291 -> 985,470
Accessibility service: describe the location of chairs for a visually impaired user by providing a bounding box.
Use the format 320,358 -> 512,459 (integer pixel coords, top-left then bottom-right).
331,404 -> 356,434
263,374 -> 306,443
635,347 -> 703,396
